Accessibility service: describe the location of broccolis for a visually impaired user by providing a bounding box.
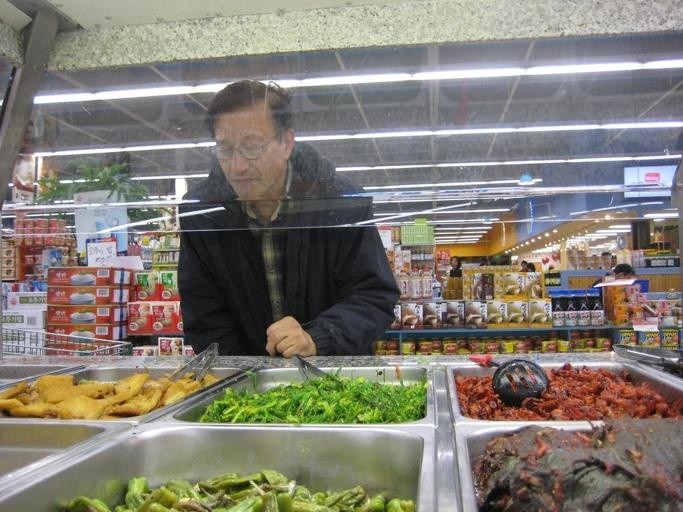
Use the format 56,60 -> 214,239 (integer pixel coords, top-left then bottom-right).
199,373 -> 427,423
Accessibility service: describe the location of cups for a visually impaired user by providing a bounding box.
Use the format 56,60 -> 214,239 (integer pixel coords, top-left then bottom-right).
553,310 -> 606,326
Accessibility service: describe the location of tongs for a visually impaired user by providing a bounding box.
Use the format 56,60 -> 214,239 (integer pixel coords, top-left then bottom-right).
170,341 -> 221,384
292,352 -> 345,389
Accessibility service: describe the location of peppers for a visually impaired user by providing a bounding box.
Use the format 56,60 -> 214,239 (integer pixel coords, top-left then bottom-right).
60,470 -> 414,512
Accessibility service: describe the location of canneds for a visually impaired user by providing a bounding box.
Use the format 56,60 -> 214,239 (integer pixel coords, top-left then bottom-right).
605,286 -> 682,350
372,331 -> 611,355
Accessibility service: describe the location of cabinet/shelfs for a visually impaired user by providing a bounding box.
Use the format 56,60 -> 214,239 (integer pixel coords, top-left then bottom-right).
370,216 -> 682,359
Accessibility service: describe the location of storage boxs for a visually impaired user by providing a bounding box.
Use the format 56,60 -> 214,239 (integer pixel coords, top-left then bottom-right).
0,213 -> 197,358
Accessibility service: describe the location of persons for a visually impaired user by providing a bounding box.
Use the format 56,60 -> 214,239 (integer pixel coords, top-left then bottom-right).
450,256 -> 462,277
520,261 -> 529,272
593,264 -> 636,286
527,263 -> 535,272
177,80 -> 401,357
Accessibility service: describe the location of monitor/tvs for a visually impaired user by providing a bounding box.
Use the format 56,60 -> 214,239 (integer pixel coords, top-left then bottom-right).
620,162 -> 679,201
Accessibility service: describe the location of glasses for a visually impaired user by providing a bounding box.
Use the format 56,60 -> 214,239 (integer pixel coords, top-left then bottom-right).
210,131 -> 280,161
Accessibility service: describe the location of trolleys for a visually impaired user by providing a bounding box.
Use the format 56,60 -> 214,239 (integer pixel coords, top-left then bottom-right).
0,325 -> 132,357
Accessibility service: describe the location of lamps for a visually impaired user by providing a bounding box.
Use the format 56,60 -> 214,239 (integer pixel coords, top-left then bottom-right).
1,51 -> 683,223
